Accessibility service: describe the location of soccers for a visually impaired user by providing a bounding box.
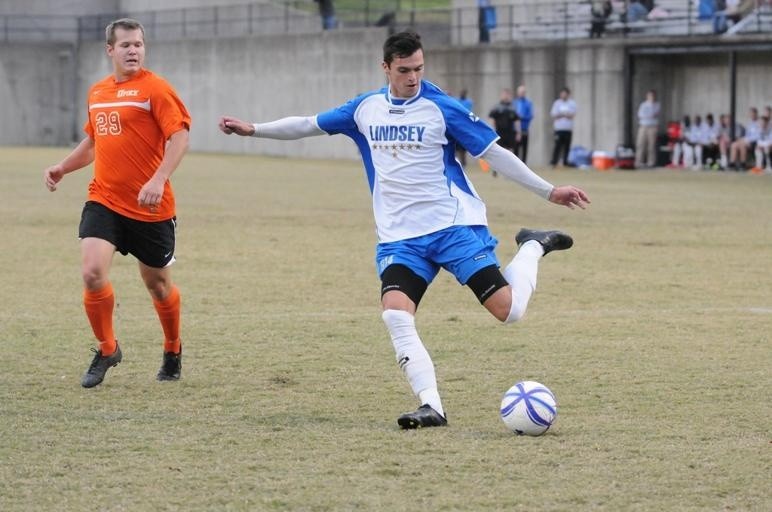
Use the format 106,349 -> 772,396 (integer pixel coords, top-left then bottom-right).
500,380 -> 555,434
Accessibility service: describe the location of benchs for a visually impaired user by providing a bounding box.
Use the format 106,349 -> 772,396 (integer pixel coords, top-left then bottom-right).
655,132 -> 772,169
508,0 -> 771,45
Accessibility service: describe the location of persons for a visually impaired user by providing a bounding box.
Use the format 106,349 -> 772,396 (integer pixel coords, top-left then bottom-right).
442,84 -> 533,174
665,104 -> 772,174
219,31 -> 589,427
314,1 -> 337,31
550,87 -> 577,172
45,19 -> 192,389
476,1 -> 492,44
633,89 -> 662,167
590,0 -> 758,39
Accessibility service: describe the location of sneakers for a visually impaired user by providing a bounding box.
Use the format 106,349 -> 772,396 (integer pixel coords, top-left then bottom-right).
514,228 -> 574,257
157,342 -> 183,382
397,404 -> 447,429
81,339 -> 122,388
